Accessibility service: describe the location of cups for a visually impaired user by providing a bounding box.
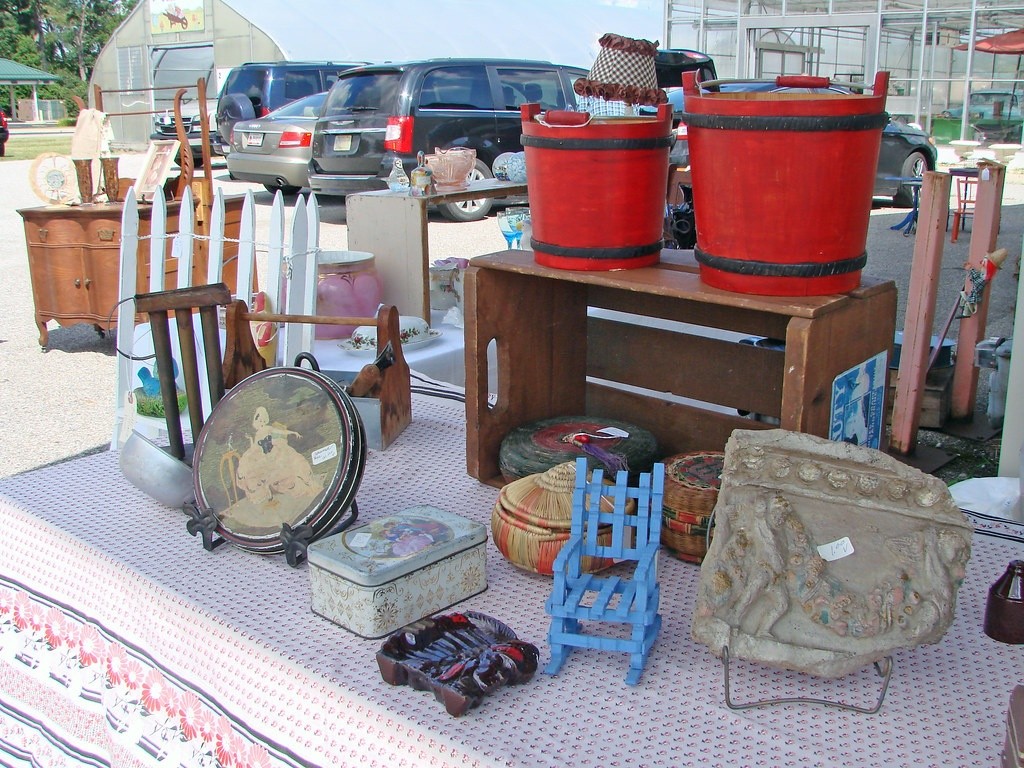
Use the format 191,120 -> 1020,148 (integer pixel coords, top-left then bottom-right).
72,158 -> 98,207
100,157 -> 122,205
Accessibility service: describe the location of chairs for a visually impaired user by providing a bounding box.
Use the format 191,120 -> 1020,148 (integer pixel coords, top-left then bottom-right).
951,178 -> 979,242
542,457 -> 665,685
524,84 -> 543,104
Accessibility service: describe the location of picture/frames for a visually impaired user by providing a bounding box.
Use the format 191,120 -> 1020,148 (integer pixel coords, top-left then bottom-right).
132,139 -> 182,204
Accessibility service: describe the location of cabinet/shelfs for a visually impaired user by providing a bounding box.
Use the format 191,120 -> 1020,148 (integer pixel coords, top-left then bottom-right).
16,190 -> 257,349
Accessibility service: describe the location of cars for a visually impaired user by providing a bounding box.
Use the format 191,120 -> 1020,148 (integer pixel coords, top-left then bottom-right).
226,92 -> 330,196
0,107 -> 10,157
654,49 -> 937,250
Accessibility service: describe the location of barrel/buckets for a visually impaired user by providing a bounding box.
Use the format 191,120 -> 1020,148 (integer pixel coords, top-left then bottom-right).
520,102 -> 676,270
682,68 -> 890,296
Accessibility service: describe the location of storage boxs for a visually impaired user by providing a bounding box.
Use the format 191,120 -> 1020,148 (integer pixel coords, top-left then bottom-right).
306,504 -> 490,640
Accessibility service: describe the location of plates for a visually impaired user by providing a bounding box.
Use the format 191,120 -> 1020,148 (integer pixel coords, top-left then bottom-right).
337,330 -> 444,358
29,152 -> 77,204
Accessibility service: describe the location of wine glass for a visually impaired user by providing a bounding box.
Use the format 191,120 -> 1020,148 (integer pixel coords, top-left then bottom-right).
497,206 -> 531,250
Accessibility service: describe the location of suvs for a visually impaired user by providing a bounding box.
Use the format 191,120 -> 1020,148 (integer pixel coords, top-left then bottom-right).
149,98 -> 223,168
211,61 -> 374,155
941,90 -> 1024,120
308,55 -> 590,222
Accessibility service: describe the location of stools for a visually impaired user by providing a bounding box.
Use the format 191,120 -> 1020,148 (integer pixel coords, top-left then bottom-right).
987,143 -> 1021,163
948,140 -> 980,156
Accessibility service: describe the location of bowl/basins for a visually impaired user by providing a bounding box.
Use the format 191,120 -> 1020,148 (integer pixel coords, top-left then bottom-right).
120,429 -> 196,509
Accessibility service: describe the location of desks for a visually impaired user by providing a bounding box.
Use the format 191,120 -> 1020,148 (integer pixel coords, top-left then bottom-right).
464,247 -> 899,507
343,177 -> 529,330
946,168 -> 980,234
884,177 -> 925,235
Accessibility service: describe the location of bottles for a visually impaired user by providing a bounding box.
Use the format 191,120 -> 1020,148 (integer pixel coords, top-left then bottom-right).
428,266 -> 466,313
